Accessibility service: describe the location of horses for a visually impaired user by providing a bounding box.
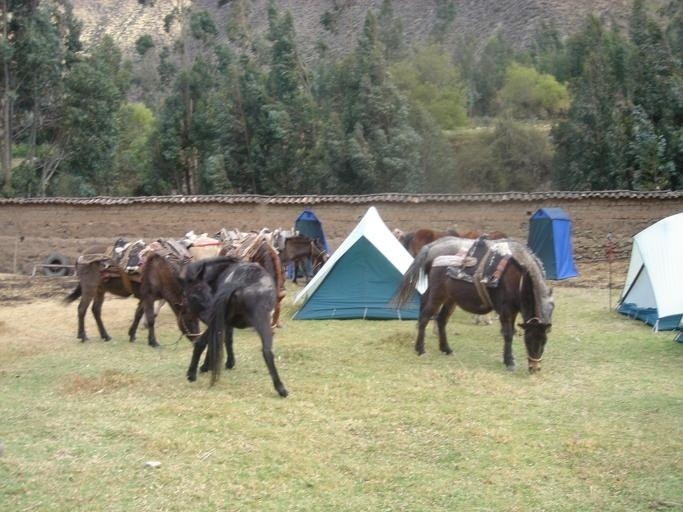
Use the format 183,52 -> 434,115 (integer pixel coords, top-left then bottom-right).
382,236 -> 556,339
399,225 -> 510,257
175,254 -> 288,398
413,250 -> 553,374
59,227 -> 330,349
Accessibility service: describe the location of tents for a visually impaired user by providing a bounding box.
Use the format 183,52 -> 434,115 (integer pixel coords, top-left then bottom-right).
528,208 -> 580,280
287,206 -> 443,320
616,213 -> 683,331
